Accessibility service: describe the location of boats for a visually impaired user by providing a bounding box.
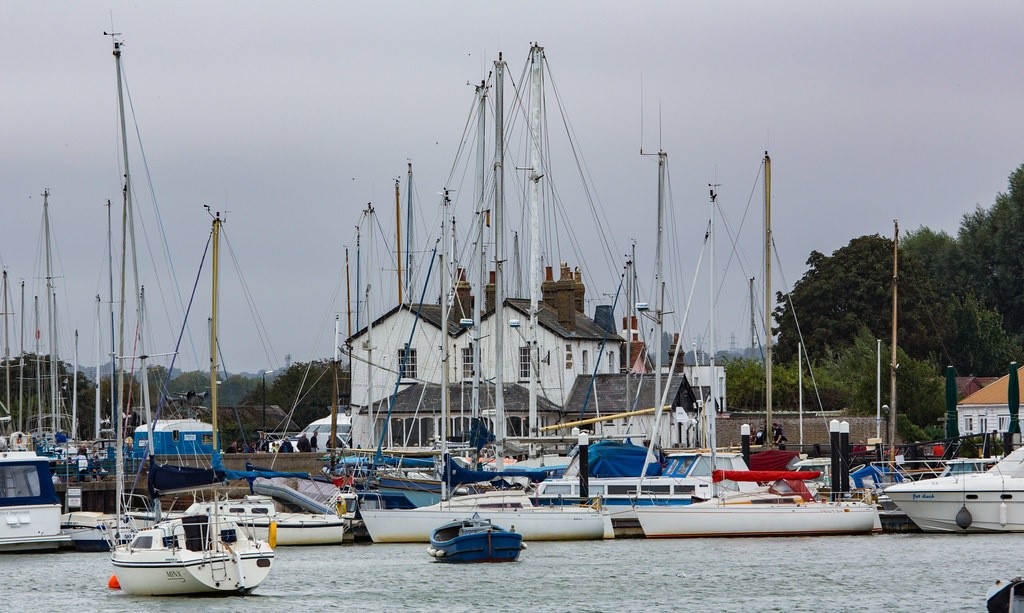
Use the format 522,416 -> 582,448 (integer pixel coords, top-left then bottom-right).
985,578 -> 1024,613
98,514 -> 275,597
427,513 -> 523,563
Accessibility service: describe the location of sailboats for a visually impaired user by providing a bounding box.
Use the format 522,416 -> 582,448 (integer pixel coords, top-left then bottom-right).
0,12 -> 1023,536
